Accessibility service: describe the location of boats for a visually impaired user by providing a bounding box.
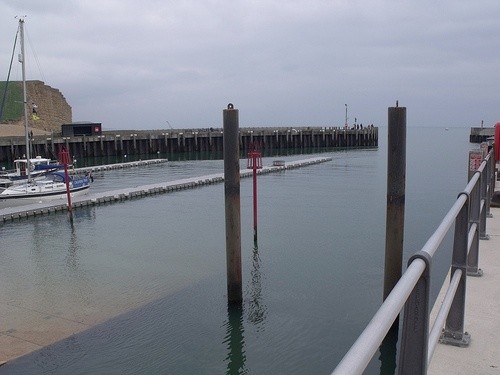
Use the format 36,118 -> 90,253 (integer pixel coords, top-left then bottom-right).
0,127 -> 64,187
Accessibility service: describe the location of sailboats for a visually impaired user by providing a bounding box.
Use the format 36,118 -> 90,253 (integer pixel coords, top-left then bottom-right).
0,13 -> 94,199
